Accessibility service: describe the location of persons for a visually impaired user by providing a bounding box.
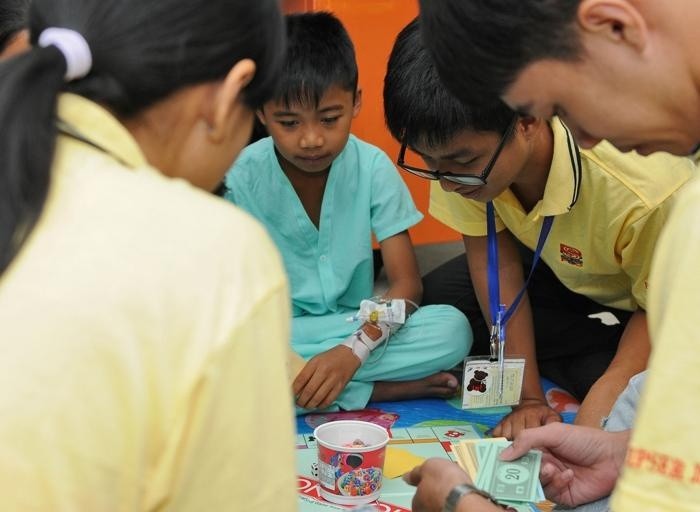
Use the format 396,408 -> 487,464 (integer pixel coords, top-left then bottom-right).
404,0 -> 700,512
384,23 -> 698,437
0,0 -> 295,512
219,11 -> 474,416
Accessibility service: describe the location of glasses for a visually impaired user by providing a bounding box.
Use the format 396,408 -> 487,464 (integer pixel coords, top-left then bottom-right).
398,115 -> 516,185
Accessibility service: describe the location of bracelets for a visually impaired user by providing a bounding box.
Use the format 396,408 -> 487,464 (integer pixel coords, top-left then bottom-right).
445,482 -> 496,511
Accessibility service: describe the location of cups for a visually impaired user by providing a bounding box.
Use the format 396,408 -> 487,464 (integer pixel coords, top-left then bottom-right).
312,420 -> 390,505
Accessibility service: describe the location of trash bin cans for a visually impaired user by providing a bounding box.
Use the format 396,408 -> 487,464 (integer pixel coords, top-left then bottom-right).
496,304 -> 506,405
